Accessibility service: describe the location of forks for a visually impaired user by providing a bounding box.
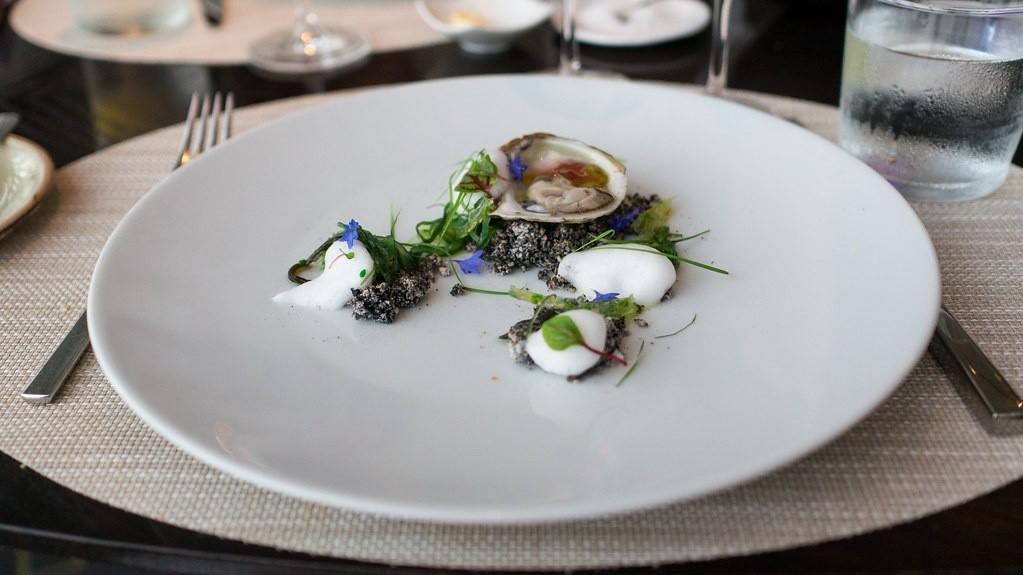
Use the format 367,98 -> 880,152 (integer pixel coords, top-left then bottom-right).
22,94 -> 233,406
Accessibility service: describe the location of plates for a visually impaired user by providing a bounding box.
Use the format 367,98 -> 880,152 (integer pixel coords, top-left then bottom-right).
552,1 -> 711,47
416,0 -> 553,53
0,135 -> 52,237
87,77 -> 941,522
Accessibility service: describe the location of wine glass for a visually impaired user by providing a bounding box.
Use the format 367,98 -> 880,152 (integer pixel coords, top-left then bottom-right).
246,0 -> 371,70
701,0 -> 801,125
522,2 -> 626,79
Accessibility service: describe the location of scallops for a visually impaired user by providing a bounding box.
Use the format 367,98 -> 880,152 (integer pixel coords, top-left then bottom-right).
489,132 -> 627,224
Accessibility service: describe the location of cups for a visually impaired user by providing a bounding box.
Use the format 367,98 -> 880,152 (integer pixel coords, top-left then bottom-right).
67,1 -> 191,41
838,1 -> 1023,198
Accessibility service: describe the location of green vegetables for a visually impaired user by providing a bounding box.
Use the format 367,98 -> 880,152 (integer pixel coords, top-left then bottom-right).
301,146 -> 729,387
